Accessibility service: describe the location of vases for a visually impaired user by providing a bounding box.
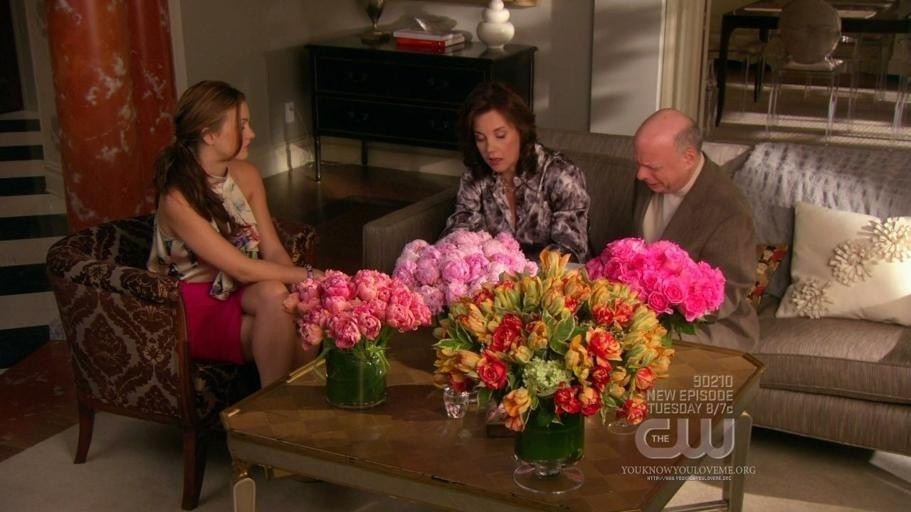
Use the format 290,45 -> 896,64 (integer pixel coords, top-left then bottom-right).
511,378 -> 586,493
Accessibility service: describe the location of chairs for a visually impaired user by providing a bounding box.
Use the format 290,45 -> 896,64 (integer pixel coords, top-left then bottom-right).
765,0 -> 911,135
45,215 -> 320,512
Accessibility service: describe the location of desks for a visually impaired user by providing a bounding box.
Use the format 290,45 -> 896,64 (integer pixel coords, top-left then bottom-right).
714,2 -> 911,129
305,34 -> 540,182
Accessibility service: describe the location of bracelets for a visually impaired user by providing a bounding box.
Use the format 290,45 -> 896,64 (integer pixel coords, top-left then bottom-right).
304,263 -> 314,280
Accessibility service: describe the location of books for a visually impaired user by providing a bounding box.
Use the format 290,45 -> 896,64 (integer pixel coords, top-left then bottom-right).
396,43 -> 465,52
396,37 -> 465,46
393,27 -> 464,41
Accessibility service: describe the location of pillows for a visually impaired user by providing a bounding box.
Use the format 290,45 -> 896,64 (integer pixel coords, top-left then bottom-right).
776,199 -> 911,324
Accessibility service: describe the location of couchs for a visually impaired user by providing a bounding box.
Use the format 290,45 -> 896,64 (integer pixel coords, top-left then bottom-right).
362,129 -> 911,458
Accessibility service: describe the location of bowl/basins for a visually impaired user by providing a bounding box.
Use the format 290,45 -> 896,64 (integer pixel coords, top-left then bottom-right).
413,17 -> 457,33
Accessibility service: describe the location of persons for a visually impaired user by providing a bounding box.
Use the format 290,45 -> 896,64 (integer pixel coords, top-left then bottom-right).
434,80 -> 593,263
603,107 -> 761,355
145,80 -> 325,482
744,245 -> 785,305
